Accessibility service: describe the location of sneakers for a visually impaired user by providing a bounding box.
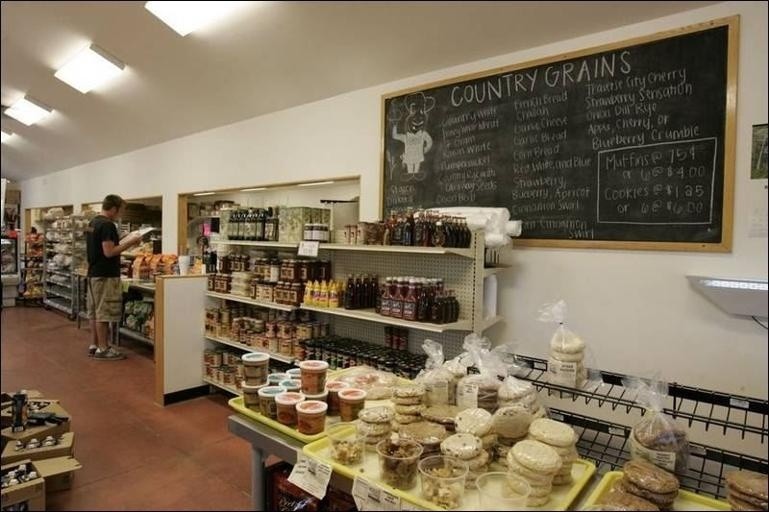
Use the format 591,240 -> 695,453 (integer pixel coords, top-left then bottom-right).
87,345 -> 129,361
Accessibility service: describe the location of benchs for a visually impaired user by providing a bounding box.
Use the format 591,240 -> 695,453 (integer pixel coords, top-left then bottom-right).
71,268 -> 127,343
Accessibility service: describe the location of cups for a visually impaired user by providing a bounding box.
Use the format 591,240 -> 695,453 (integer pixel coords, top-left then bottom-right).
326,420 -> 369,463
417,453 -> 470,508
376,438 -> 422,488
476,472 -> 530,510
177,255 -> 191,276
240,351 -> 365,435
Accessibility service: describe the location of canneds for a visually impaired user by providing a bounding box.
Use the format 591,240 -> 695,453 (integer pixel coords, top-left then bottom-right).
203,221 -> 428,435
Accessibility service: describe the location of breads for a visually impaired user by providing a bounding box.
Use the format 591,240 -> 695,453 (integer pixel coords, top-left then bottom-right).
724,470 -> 768,511
356,332 -> 583,509
600,423 -> 689,512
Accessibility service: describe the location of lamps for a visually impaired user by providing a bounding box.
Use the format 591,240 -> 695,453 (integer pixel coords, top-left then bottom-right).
54,43 -> 124,93
144,1 -> 202,36
3,94 -> 52,126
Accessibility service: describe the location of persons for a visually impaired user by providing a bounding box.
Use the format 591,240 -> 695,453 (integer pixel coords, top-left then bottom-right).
86,194 -> 143,361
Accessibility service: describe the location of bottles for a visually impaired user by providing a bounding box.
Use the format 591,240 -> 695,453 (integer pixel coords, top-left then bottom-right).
227,206 -> 280,241
304,223 -> 329,243
302,272 -> 459,324
366,205 -> 471,248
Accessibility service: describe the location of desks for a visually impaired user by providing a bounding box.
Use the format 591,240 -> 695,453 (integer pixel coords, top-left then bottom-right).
116,277 -> 156,346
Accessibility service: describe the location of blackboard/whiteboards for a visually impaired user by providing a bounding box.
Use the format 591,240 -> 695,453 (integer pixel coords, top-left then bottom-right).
380,13 -> 740,252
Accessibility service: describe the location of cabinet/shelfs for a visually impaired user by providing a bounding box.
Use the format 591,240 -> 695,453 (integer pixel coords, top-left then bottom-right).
203,241 -> 511,397
23,216 -> 130,324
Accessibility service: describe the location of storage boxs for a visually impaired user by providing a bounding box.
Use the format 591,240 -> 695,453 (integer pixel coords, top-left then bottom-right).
1,387 -> 82,512
